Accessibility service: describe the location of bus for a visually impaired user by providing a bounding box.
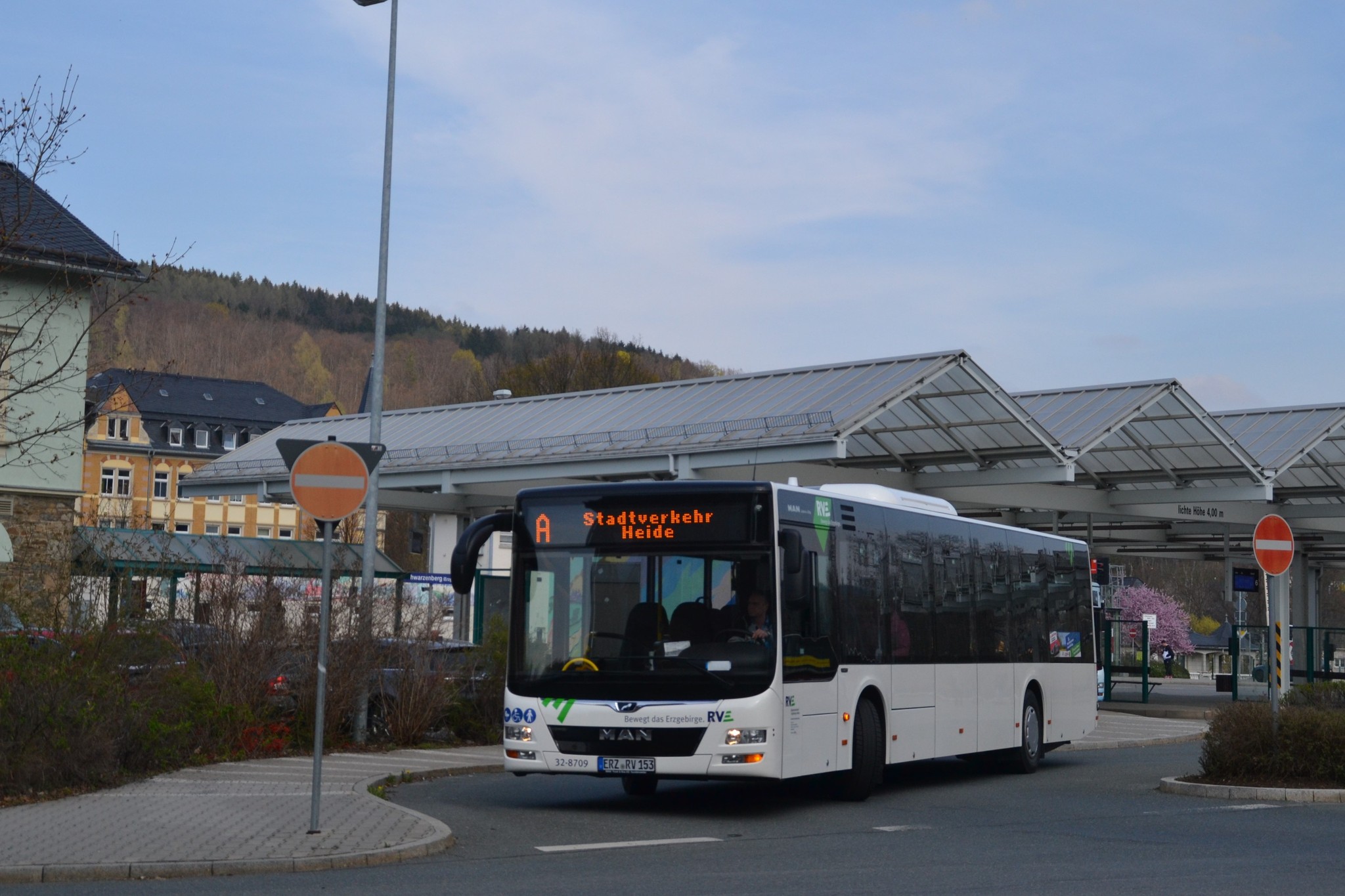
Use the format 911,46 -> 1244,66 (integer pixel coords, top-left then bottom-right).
451,476 -> 1097,799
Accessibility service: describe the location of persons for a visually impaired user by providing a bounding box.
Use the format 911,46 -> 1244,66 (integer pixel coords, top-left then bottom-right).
1161,640 -> 1174,678
300,615 -> 319,634
728,590 -> 775,652
888,575 -> 910,656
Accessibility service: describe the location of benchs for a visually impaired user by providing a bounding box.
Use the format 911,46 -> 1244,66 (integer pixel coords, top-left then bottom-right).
1111,681 -> 1161,695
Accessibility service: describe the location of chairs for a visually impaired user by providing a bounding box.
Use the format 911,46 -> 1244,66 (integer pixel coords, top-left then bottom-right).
626,602 -> 717,644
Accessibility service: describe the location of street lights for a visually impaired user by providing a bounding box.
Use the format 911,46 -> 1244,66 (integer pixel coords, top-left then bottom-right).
355,0 -> 398,751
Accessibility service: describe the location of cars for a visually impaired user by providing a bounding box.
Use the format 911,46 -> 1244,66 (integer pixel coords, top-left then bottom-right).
0,623 -> 496,742
1252,659 -> 1332,683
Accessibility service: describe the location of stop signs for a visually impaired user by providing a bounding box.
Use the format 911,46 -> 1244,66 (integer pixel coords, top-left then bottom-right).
290,441 -> 370,522
1252,514 -> 1294,576
1129,628 -> 1136,638
